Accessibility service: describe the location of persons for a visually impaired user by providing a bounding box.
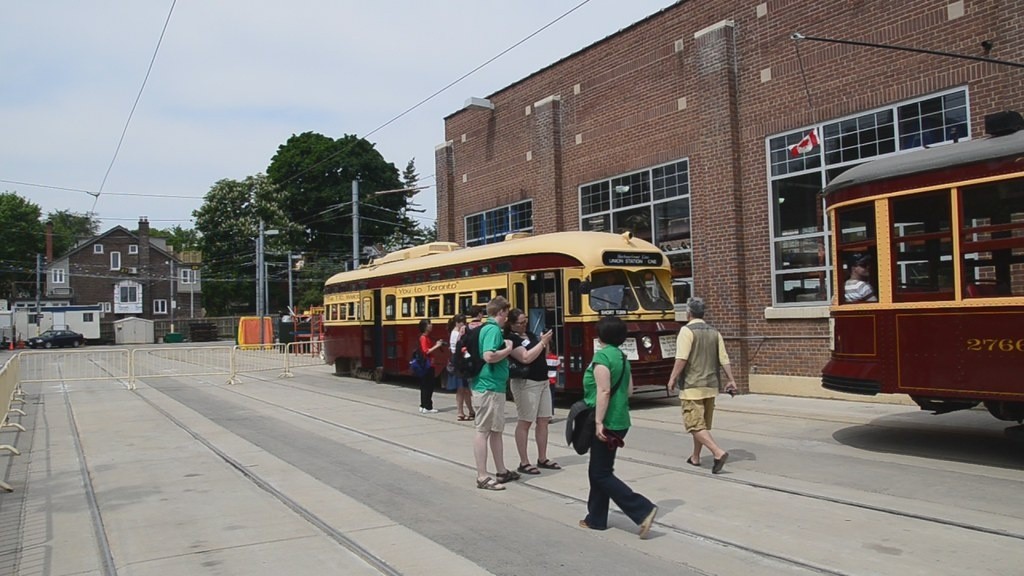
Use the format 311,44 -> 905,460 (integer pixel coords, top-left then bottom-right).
472,295 -> 522,492
591,269 -> 625,312
579,314 -> 659,540
535,338 -> 562,424
459,304 -> 486,394
841,251 -> 879,305
446,312 -> 478,420
504,306 -> 566,475
669,296 -> 739,474
417,316 -> 446,415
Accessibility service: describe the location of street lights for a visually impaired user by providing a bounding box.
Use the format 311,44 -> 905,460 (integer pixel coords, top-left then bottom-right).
288,250 -> 302,314
259,221 -> 279,317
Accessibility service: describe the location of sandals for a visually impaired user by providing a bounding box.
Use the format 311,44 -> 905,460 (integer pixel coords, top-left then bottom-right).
537,459 -> 563,470
495,471 -> 522,483
517,463 -> 541,475
468,412 -> 476,421
458,415 -> 475,422
477,479 -> 506,492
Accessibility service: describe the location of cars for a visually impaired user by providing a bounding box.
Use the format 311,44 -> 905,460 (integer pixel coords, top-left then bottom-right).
27,330 -> 84,349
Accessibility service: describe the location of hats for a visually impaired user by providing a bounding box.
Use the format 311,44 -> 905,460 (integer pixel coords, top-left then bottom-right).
847,254 -> 874,268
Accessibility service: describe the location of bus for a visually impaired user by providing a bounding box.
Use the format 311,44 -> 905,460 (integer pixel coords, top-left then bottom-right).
323,230 -> 681,403
819,111 -> 1024,422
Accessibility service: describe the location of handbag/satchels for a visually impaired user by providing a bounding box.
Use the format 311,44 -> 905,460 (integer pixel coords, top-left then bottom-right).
446,358 -> 456,374
565,350 -> 628,455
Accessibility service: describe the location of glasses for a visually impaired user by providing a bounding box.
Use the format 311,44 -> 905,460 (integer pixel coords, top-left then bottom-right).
855,262 -> 873,268
458,320 -> 466,324
510,320 -> 527,327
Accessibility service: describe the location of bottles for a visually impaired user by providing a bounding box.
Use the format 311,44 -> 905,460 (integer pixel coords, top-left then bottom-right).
464,350 -> 472,367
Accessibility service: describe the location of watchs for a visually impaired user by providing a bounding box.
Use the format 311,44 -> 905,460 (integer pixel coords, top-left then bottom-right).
541,340 -> 547,347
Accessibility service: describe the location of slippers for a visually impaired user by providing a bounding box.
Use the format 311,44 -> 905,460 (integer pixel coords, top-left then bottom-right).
688,455 -> 701,466
711,453 -> 729,473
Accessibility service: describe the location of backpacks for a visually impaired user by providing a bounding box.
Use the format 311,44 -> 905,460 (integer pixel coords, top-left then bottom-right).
409,344 -> 431,379
453,321 -> 508,377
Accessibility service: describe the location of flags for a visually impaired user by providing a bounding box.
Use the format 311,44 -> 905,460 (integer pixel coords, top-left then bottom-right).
788,127 -> 820,158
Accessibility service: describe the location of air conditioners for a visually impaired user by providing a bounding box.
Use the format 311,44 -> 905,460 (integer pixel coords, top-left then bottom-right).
128,268 -> 136,273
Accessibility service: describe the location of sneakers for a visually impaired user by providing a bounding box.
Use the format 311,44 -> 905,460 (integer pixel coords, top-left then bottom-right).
423,407 -> 438,414
419,406 -> 424,413
578,518 -> 588,528
638,506 -> 658,539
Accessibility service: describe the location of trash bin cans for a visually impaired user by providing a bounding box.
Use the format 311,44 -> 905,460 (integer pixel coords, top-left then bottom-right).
113,317 -> 154,343
279,322 -> 311,355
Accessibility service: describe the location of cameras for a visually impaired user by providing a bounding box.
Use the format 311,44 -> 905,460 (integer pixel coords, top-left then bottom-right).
441,341 -> 449,347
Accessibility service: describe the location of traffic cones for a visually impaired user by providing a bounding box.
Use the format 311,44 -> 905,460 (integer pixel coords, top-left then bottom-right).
9,341 -> 14,350
